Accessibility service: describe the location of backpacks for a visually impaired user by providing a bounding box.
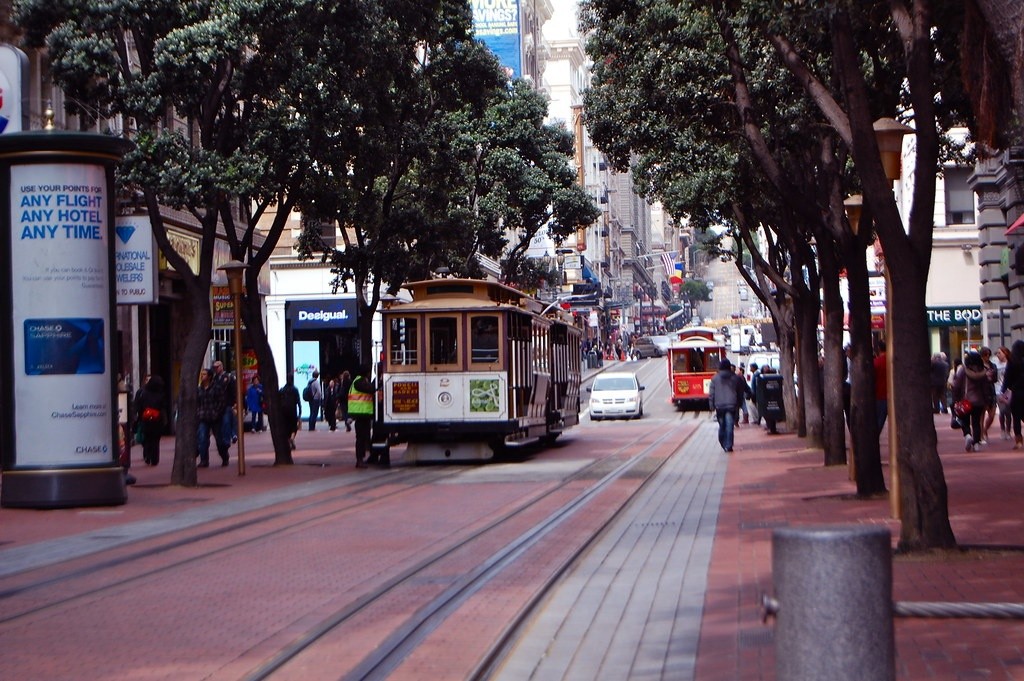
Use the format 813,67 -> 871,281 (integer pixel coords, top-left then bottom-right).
303,379 -> 317,401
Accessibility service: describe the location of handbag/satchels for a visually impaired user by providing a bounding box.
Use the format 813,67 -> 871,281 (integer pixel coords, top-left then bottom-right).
142,408 -> 160,420
954,400 -> 972,415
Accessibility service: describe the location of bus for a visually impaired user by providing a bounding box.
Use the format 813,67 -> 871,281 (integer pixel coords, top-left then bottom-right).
666,326 -> 732,410
371,267 -> 594,462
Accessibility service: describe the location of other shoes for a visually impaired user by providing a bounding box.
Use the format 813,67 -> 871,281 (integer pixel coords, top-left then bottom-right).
941,407 -> 947,413
721,443 -> 733,452
222,457 -> 229,466
973,443 -> 980,451
735,412 -> 761,426
309,420 -> 353,433
1000,431 -> 1012,441
980,432 -> 992,446
232,436 -> 237,444
964,434 -> 974,451
197,458 -> 209,467
1014,435 -> 1022,450
934,407 -> 940,413
288,439 -> 296,450
144,455 -> 159,466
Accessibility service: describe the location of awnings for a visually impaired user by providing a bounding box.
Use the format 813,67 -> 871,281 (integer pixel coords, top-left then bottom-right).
582,263 -> 598,283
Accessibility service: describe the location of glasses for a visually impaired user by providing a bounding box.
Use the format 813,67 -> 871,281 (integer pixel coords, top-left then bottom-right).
985,353 -> 992,356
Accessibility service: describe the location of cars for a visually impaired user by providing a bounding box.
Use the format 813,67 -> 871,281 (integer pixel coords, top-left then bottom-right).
631,336 -> 672,360
718,324 -> 825,354
585,372 -> 645,421
743,352 -> 797,389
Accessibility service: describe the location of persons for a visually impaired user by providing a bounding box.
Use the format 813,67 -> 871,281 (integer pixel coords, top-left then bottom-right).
322,371 -> 354,432
730,362 -> 777,424
472,325 -> 497,361
709,358 -> 744,452
628,329 -> 667,349
348,363 -> 382,469
133,375 -> 168,466
197,361 -> 265,467
931,339 -> 1024,452
280,385 -> 302,451
692,348 -> 704,371
843,339 -> 888,494
308,371 -> 321,431
580,335 -> 623,367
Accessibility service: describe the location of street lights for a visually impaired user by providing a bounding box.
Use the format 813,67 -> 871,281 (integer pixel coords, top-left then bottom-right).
866,117 -> 915,552
216,260 -> 251,475
693,249 -> 702,274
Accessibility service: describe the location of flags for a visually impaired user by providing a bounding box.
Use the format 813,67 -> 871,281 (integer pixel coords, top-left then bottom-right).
661,252 -> 679,275
669,263 -> 685,284
